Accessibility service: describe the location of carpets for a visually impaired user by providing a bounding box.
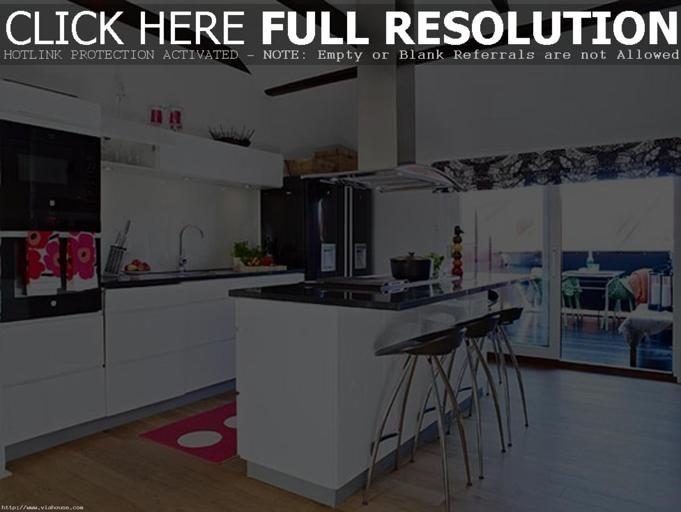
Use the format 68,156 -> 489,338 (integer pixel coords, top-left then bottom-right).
141,401 -> 241,464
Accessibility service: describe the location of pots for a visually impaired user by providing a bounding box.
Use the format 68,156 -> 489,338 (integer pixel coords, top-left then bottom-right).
389,251 -> 431,283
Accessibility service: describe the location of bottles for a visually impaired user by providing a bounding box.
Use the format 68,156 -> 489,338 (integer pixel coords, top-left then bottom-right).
148,104 -> 185,132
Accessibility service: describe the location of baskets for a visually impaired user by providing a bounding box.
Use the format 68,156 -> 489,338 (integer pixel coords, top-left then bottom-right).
284,156 -> 316,175
311,147 -> 356,172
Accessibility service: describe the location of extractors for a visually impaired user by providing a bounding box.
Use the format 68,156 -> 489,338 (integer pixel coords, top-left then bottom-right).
300,0 -> 465,194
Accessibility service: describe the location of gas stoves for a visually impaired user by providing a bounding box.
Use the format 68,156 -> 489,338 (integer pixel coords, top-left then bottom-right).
301,274 -> 461,289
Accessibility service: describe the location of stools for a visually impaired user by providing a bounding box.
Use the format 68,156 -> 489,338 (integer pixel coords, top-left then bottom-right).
362,305 -> 529,511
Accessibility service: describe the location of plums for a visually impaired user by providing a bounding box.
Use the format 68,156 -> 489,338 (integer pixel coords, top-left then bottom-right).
451,234 -> 463,286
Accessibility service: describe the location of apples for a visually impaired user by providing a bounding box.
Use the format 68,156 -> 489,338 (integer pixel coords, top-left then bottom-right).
142,263 -> 151,271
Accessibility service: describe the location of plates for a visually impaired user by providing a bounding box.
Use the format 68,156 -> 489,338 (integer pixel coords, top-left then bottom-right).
121,271 -> 154,275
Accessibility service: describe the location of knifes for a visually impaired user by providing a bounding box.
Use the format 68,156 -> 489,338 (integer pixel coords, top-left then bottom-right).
115,219 -> 132,248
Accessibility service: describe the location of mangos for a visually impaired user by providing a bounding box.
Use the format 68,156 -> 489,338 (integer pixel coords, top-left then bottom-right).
126,263 -> 138,271
138,264 -> 144,271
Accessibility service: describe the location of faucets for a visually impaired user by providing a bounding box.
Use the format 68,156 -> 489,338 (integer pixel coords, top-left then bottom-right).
178,223 -> 204,258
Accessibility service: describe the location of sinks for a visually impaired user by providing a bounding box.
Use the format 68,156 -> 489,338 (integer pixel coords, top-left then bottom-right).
122,267 -> 181,275
184,267 -> 233,271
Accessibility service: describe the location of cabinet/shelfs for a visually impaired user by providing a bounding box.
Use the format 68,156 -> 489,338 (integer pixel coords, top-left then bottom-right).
1,312 -> 104,461
107,274 -> 307,430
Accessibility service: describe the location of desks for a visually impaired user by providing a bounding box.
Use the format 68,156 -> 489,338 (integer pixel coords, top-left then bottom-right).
561,268 -> 628,332
623,304 -> 674,367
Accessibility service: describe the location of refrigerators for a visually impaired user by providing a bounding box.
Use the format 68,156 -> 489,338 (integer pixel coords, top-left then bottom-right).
260,178 -> 375,283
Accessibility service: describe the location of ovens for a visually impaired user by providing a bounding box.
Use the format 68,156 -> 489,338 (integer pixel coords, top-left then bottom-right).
0,118 -> 102,324
